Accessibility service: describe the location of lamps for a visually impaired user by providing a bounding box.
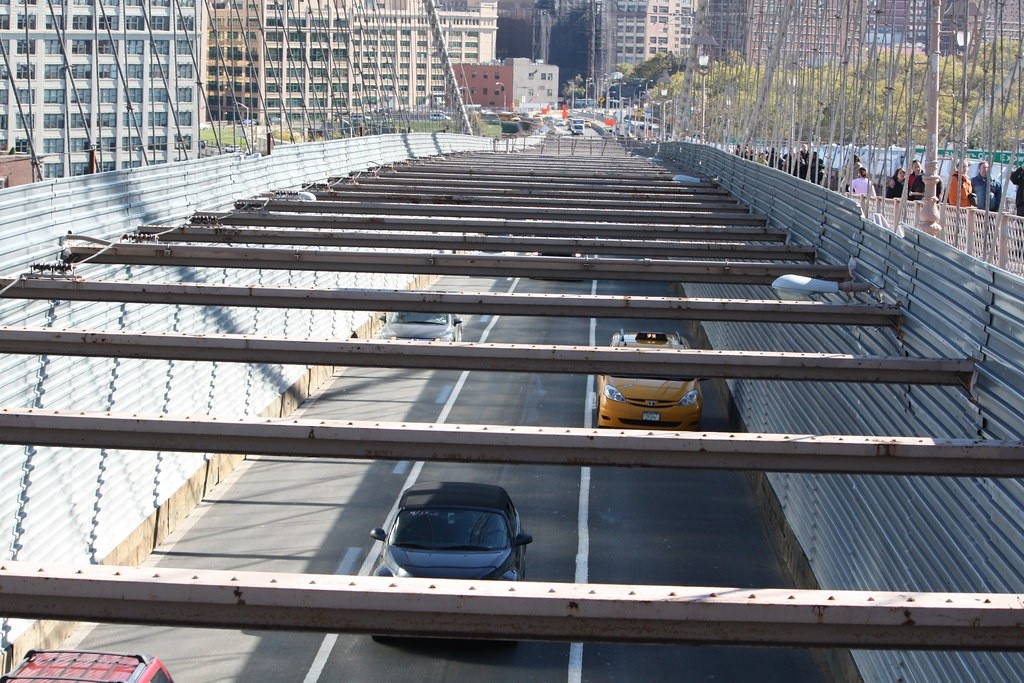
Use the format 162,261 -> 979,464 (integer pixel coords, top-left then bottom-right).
771,273 -> 877,299
673,175 -> 722,185
647,157 -> 672,163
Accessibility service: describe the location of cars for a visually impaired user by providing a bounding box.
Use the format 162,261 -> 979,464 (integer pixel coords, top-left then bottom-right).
318,120 -> 352,136
584,120 -> 591,128
499,112 -> 564,127
266,118 -> 285,125
225,144 -> 243,153
199,123 -> 211,130
429,113 -> 451,121
596,330 -> 702,429
368,482 -> 533,643
640,123 -> 652,130
246,145 -> 257,154
351,114 -> 371,122
198,141 -> 205,148
239,117 -> 257,125
377,311 -> 463,343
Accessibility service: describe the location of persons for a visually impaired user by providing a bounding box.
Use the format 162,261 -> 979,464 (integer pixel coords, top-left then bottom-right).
948,160 -> 972,206
1010,162 -> 1024,260
886,169 -> 907,199
848,168 -> 876,197
846,155 -> 863,192
971,161 -> 1002,211
736,143 -> 825,185
909,159 -> 924,201
909,167 -> 946,203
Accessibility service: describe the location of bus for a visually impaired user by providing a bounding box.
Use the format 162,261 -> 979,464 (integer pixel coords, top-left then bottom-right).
463,105 -> 481,120
470,112 -> 501,138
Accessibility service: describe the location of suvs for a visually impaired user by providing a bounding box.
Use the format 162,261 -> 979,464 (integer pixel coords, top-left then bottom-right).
0,648 -> 172,683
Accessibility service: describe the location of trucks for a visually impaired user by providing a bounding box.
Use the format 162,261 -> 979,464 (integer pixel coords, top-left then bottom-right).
570,119 -> 585,136
566,116 -> 574,125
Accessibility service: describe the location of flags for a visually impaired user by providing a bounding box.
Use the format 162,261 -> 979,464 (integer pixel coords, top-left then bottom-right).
542,109 -> 547,114
605,119 -> 615,126
562,111 -> 567,118
562,104 -> 567,110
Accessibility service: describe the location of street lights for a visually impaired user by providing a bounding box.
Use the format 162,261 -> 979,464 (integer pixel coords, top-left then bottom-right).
585,74 -> 616,120
787,74 -> 798,147
460,86 -> 466,98
633,79 -> 673,141
495,83 -> 507,111
235,102 -> 248,127
658,74 -> 672,141
567,80 -> 574,111
956,17 -> 968,157
618,82 -> 627,123
693,34 -> 718,142
